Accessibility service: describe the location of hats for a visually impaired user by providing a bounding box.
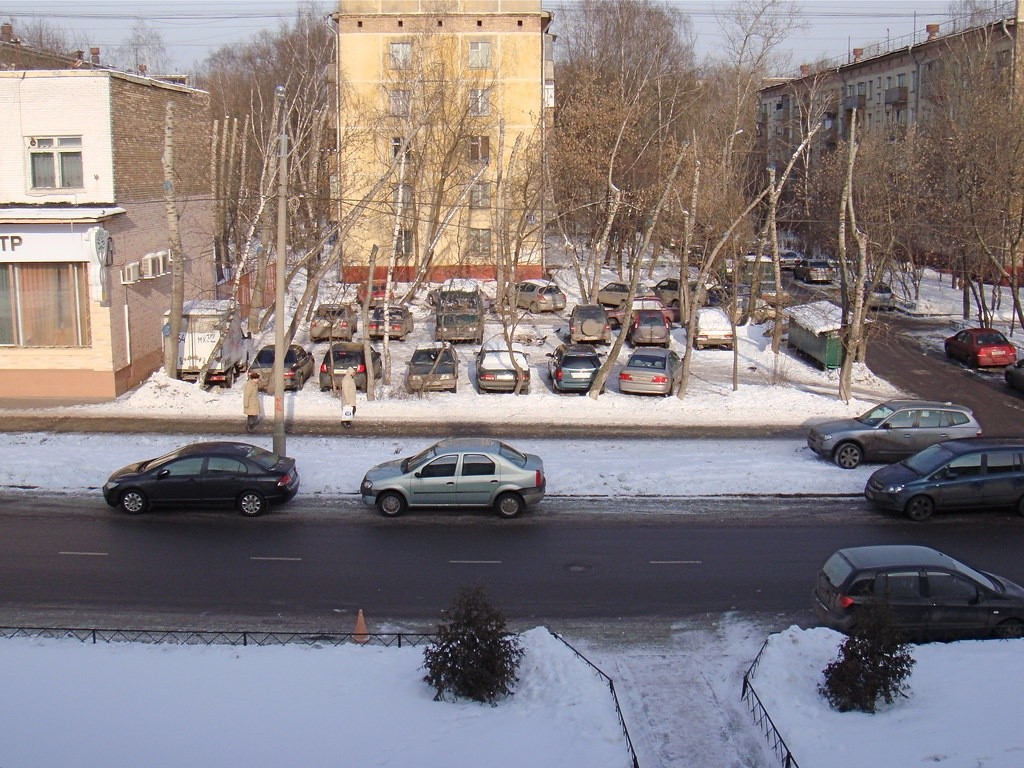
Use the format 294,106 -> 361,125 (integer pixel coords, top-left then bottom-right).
250,373 -> 259,378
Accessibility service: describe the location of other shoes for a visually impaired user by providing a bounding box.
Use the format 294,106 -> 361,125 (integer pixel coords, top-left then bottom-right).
347,424 -> 351,428
246,426 -> 251,432
251,427 -> 255,432
341,422 -> 347,428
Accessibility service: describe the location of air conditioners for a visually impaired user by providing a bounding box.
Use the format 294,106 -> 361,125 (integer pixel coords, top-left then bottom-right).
126,250 -> 169,281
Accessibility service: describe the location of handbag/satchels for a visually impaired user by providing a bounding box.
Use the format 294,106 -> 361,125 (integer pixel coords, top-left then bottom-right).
342,403 -> 354,421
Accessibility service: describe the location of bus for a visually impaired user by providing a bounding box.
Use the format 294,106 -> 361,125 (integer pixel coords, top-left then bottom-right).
435,278 -> 485,346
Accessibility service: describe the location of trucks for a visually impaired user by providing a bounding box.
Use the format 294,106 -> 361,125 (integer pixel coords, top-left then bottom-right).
160,298 -> 251,388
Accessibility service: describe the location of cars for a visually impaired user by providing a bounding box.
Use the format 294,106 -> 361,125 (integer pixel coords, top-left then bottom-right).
859,281 -> 896,311
367,305 -> 414,341
318,342 -> 383,393
684,307 -> 734,350
426,287 -> 491,311
618,347 -> 684,397
248,343 -> 316,391
706,283 -> 757,309
101,441 -> 301,517
630,312 -> 670,349
362,437 -> 547,518
944,328 -> 1017,369
474,342 -> 531,395
864,436 -> 1023,523
546,343 -> 606,394
310,303 -> 358,342
504,279 -> 567,314
605,297 -> 676,328
355,279 -> 395,311
1004,358 -> 1024,392
792,258 -> 836,284
405,341 -> 461,393
779,251 -> 801,270
649,278 -> 711,313
597,281 -> 657,309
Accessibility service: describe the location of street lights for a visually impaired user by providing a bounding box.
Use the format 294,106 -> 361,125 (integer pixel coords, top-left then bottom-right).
272,83 -> 290,457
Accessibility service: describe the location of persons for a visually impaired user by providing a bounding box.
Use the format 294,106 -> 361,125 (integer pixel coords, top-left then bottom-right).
244,373 -> 260,432
342,367 -> 356,428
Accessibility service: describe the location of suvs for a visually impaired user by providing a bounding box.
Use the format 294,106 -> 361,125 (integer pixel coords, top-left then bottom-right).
810,543 -> 1023,644
569,304 -> 612,347
806,397 -> 983,470
